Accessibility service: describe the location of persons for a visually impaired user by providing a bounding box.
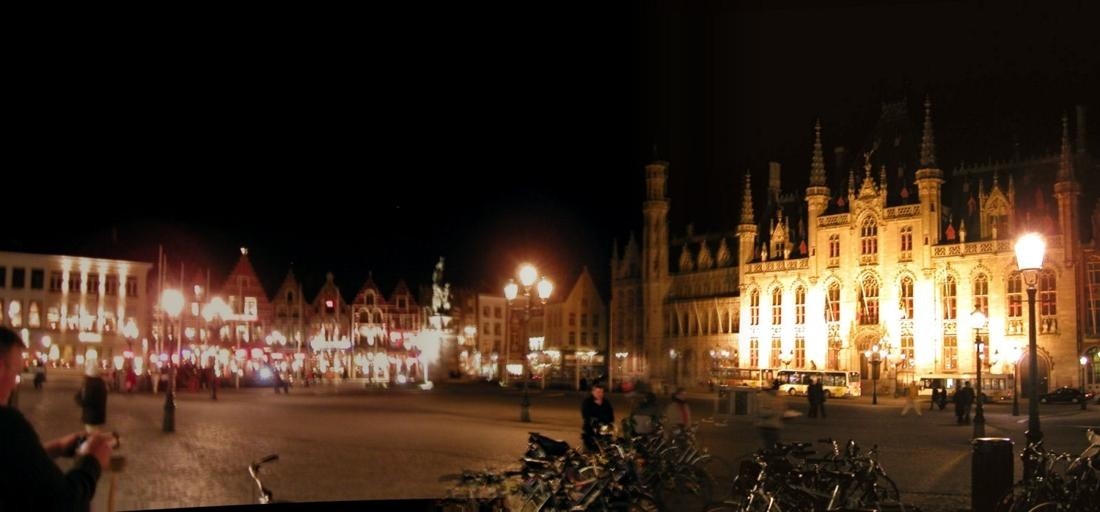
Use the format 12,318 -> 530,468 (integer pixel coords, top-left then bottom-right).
938,385 -> 948,409
962,381 -> 975,420
929,385 -> 941,411
0,321 -> 114,510
665,385 -> 692,428
900,381 -> 923,418
953,385 -> 966,422
580,382 -> 614,455
807,378 -> 817,419
431,281 -> 447,317
815,376 -> 828,420
441,281 -> 455,316
33,363 -> 45,391
74,364 -> 123,452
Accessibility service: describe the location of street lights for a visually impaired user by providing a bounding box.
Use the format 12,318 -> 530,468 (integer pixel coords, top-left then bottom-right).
503,263 -> 553,425
968,303 -> 986,440
864,344 -> 886,405
160,286 -> 187,433
202,300 -> 231,399
1014,231 -> 1046,446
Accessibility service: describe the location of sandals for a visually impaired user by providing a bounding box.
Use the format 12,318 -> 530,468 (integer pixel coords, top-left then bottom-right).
709,364 -> 863,398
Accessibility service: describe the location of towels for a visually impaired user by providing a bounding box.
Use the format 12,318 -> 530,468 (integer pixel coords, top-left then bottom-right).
971,437 -> 1014,512
717,385 -> 752,415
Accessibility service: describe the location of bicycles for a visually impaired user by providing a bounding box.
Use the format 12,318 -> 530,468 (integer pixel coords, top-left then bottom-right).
440,392 -> 901,511
248,451 -> 297,504
1002,430 -> 1100,512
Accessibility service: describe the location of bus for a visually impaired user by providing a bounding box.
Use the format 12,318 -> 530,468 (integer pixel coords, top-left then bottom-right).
709,364 -> 863,398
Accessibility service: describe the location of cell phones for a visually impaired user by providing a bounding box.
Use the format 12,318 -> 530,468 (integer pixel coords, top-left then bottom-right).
74,431 -> 120,452
